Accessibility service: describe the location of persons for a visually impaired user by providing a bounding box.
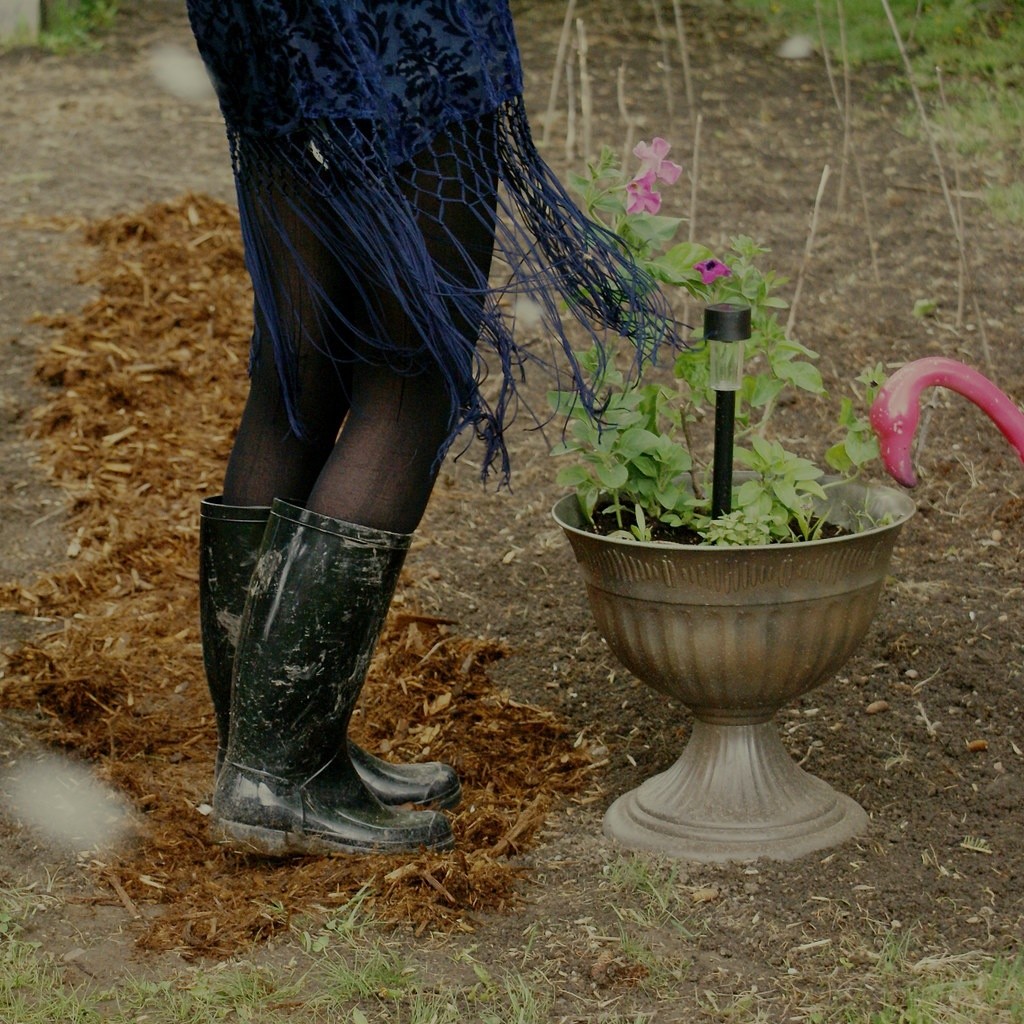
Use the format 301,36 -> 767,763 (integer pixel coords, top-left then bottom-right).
187,0 -> 526,859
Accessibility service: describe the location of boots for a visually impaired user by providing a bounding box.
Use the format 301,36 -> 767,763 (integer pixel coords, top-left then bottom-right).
205,498 -> 454,859
198,480 -> 460,810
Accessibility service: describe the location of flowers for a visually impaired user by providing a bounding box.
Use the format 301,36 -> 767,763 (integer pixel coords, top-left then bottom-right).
547,134 -> 887,547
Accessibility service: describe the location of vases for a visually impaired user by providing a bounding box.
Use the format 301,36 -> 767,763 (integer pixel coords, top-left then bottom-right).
552,469 -> 917,861
